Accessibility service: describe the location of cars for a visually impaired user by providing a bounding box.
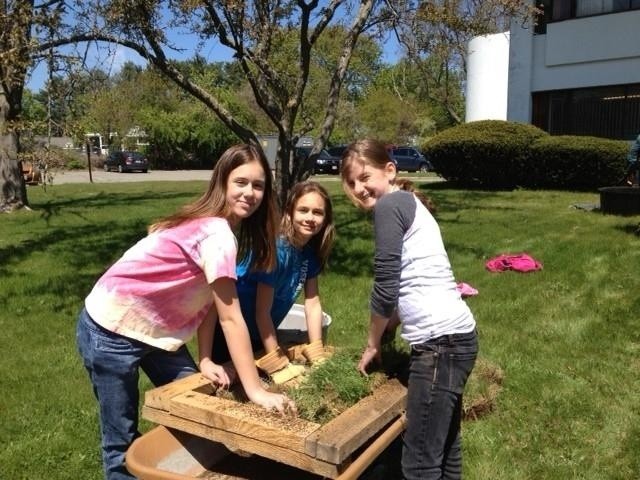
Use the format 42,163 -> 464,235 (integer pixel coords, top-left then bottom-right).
104,151 -> 148,172
293,144 -> 436,175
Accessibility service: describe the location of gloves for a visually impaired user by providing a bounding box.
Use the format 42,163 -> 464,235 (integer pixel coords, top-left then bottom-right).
252,347 -> 308,390
302,340 -> 327,368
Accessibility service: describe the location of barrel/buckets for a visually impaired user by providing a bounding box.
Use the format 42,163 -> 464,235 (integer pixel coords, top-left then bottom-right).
274,304 -> 333,347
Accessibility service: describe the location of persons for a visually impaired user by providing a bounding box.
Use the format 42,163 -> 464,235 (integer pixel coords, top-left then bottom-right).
74,144 -> 298,480
339,137 -> 478,480
212,180 -> 335,387
